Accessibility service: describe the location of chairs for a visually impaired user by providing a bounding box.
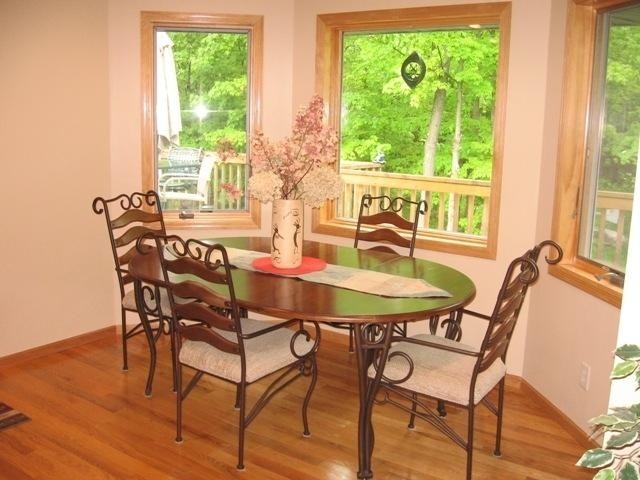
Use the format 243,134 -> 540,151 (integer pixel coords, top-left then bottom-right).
136,232 -> 321,471
313,194 -> 430,352
90,190 -> 226,394
357,239 -> 563,480
163,156 -> 216,206
157,145 -> 204,193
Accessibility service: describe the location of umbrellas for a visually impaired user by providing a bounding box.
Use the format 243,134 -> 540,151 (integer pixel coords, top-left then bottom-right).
156,30 -> 184,157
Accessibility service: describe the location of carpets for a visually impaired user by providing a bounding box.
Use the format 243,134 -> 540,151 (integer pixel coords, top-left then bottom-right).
0,401 -> 30,432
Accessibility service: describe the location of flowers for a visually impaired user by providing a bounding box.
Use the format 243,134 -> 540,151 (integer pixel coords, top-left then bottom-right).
215,93 -> 346,208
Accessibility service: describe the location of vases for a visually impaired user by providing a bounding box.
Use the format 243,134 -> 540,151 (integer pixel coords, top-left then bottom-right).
270,199 -> 305,269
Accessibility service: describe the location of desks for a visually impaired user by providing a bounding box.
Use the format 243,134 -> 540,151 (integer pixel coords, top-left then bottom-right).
127,236 -> 476,480
158,160 -> 202,207
